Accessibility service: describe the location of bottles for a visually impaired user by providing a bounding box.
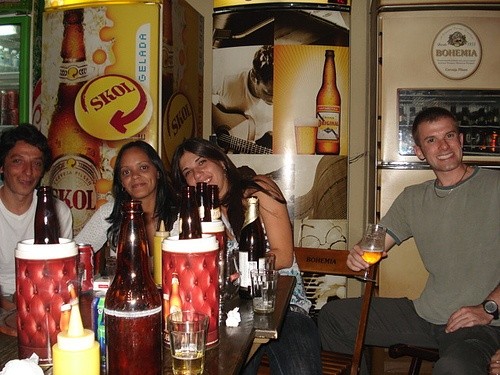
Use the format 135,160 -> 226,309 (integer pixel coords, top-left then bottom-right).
33,181 -> 265,375
46,7 -> 103,239
400,103 -> 500,157
316,50 -> 341,155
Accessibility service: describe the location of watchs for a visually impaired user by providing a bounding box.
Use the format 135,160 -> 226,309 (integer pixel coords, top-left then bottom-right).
481,299 -> 500,320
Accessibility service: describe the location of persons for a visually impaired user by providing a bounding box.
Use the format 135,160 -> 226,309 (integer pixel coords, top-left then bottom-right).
73,140 -> 176,287
171,136 -> 311,321
0,123 -> 75,312
317,107 -> 500,375
489,350 -> 500,375
211,45 -> 273,147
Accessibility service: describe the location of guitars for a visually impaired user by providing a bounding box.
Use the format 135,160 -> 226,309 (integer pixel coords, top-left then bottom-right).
211,103 -> 273,155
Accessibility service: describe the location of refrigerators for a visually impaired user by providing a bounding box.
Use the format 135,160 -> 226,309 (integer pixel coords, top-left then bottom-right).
372,0 -> 500,300
44,1 -> 204,239
0,0 -> 44,187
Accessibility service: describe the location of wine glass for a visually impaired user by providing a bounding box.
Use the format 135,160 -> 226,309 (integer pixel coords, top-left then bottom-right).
355,223 -> 386,282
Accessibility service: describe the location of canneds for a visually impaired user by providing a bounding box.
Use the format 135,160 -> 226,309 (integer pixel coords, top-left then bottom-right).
76,244 -> 94,293
90,294 -> 107,362
490,132 -> 498,152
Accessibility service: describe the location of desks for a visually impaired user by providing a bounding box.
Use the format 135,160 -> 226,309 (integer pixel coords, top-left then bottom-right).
0,275 -> 296,375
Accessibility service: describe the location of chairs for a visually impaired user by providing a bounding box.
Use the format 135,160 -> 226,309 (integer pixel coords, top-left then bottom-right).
261,247 -> 377,375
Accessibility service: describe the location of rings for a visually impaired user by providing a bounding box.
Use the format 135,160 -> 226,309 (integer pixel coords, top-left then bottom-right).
497,360 -> 498,364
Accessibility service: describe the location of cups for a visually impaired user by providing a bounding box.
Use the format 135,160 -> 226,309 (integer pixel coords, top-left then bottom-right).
163,232 -> 222,352
14,237 -> 80,366
200,221 -> 226,288
294,117 -> 317,155
166,309 -> 211,359
250,268 -> 279,314
265,253 -> 276,271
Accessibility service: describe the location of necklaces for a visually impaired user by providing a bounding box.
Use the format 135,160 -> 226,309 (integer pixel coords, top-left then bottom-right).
434,165 -> 467,198
